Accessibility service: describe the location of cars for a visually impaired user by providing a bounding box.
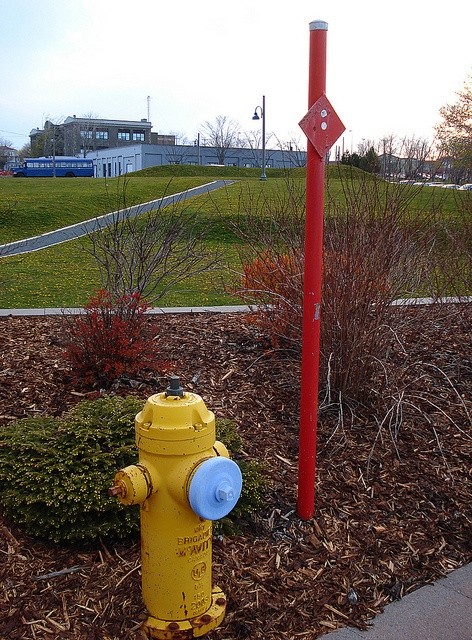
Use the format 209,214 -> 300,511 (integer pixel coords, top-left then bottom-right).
389,180 -> 472,191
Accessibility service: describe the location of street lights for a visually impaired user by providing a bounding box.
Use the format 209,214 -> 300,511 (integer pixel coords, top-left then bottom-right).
252,96 -> 267,181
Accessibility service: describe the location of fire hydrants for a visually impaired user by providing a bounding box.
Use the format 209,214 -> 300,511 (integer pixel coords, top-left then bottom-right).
109,376 -> 242,640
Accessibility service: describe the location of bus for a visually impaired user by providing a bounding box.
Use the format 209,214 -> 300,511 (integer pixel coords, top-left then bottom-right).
11,157 -> 94,177
